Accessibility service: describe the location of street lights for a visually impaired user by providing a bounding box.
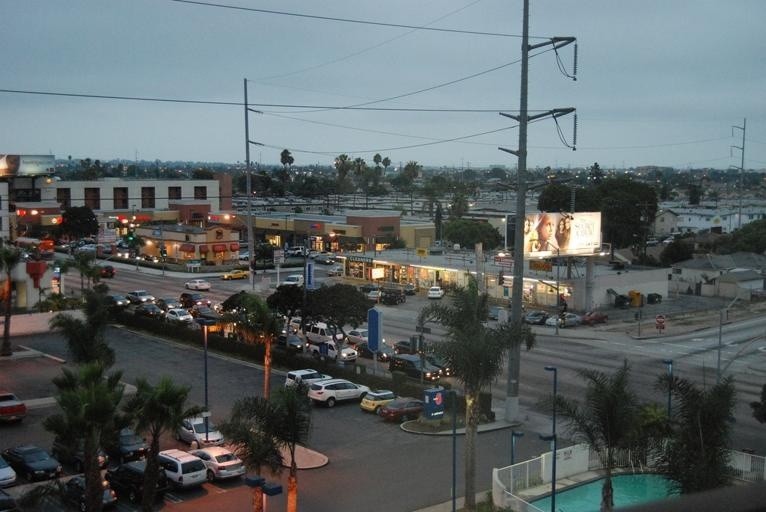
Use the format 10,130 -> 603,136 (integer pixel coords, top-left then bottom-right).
542,364 -> 559,512
418,386 -> 457,511
509,427 -> 522,466
660,357 -> 674,436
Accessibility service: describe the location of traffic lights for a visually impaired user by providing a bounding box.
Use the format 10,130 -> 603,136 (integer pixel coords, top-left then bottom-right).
128,233 -> 133,242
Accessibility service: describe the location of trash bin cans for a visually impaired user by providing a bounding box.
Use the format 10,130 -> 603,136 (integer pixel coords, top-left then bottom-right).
628,289 -> 641,306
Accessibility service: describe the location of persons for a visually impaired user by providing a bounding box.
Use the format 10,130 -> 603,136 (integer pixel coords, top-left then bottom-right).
563,215 -> 575,248
555,219 -> 566,251
524,216 -> 533,236
528,213 -> 555,252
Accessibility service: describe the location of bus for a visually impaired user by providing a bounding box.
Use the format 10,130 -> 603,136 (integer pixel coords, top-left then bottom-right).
12,236 -> 53,263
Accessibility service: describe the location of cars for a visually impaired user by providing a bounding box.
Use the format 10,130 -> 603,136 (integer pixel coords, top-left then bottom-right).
53,234 -> 112,282
115,235 -> 176,266
181,259 -> 204,270
640,230 -> 677,248
236,238 -> 348,276
0,388 -> 247,511
481,304 -> 615,329
222,269 -> 249,280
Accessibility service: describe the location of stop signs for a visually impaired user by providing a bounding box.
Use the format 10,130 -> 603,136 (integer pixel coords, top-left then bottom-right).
655,315 -> 665,325
